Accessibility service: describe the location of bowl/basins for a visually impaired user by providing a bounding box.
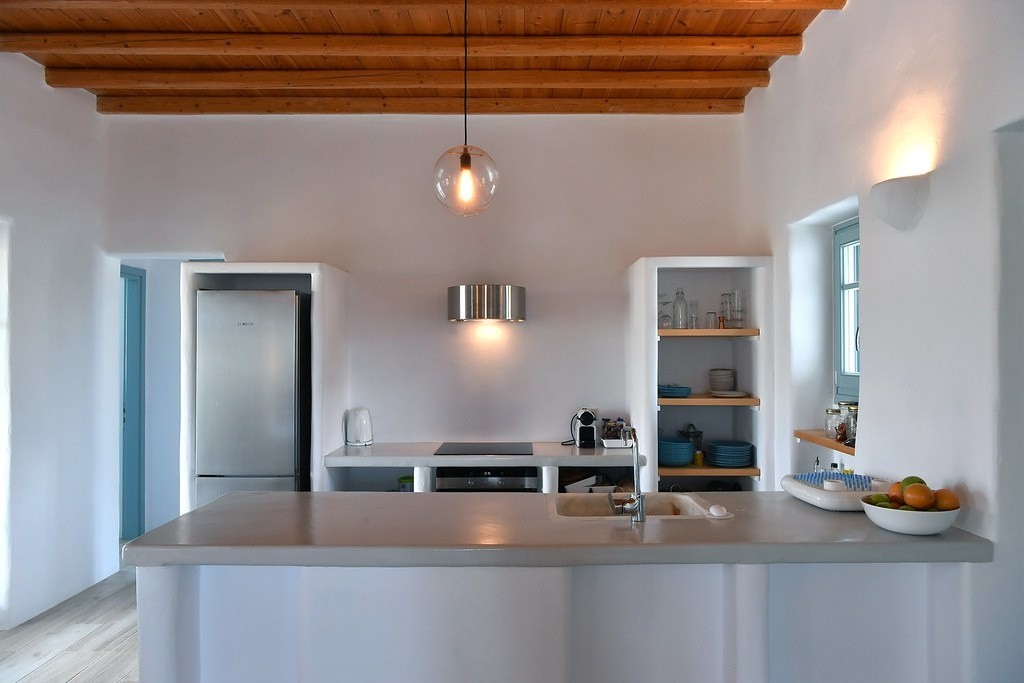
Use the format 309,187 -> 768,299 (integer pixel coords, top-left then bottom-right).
708,368 -> 734,391
860,493 -> 961,535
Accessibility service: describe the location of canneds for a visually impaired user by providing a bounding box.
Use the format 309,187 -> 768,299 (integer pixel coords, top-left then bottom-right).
606,423 -> 617,439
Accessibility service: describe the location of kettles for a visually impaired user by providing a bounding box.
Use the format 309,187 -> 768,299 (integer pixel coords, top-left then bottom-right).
347,407 -> 373,445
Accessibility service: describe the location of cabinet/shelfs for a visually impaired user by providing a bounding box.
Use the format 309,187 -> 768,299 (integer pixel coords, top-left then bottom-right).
626,255 -> 776,492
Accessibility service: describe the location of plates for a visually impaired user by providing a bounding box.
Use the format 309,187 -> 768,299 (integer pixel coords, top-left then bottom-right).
658,385 -> 692,397
658,439 -> 694,467
704,441 -> 754,467
708,390 -> 749,398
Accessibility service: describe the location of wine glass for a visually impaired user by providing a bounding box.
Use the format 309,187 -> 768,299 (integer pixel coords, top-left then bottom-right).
658,302 -> 673,328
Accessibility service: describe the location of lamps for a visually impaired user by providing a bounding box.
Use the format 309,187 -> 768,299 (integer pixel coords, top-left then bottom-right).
432,0 -> 499,217
447,284 -> 526,323
869,175 -> 928,231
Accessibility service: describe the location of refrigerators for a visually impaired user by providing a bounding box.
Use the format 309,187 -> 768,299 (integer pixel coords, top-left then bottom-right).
196,288 -> 307,508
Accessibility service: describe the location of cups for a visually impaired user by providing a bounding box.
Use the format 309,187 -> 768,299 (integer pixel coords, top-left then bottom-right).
871,479 -> 893,492
689,301 -> 700,329
843,469 -> 853,473
823,480 -> 845,492
721,289 -> 745,328
704,311 -> 718,329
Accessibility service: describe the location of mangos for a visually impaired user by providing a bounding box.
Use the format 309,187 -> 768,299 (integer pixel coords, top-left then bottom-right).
870,476 -> 959,512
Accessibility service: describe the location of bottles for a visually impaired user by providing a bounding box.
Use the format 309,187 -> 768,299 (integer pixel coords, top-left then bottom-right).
718,316 -> 725,329
825,408 -> 840,438
845,407 -> 858,439
673,287 -> 688,329
838,401 -> 858,424
827,463 -> 841,473
602,416 -> 633,440
813,457 -> 820,472
695,451 -> 703,466
